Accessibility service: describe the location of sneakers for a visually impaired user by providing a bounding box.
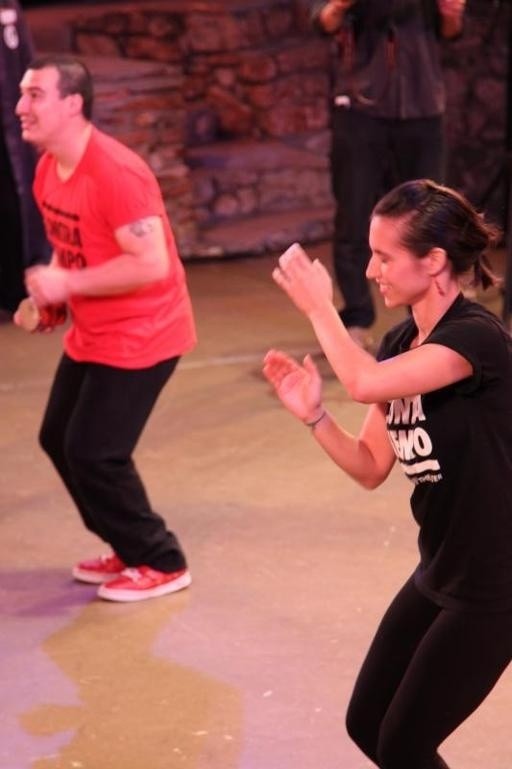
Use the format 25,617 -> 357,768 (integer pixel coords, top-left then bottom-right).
71,552 -> 192,601
336,304 -> 374,329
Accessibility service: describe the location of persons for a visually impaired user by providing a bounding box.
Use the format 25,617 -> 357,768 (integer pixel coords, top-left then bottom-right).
12,58 -> 196,603
260,177 -> 512,767
310,1 -> 466,328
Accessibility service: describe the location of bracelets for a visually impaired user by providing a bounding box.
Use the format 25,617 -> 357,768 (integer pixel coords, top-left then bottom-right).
305,411 -> 325,427
